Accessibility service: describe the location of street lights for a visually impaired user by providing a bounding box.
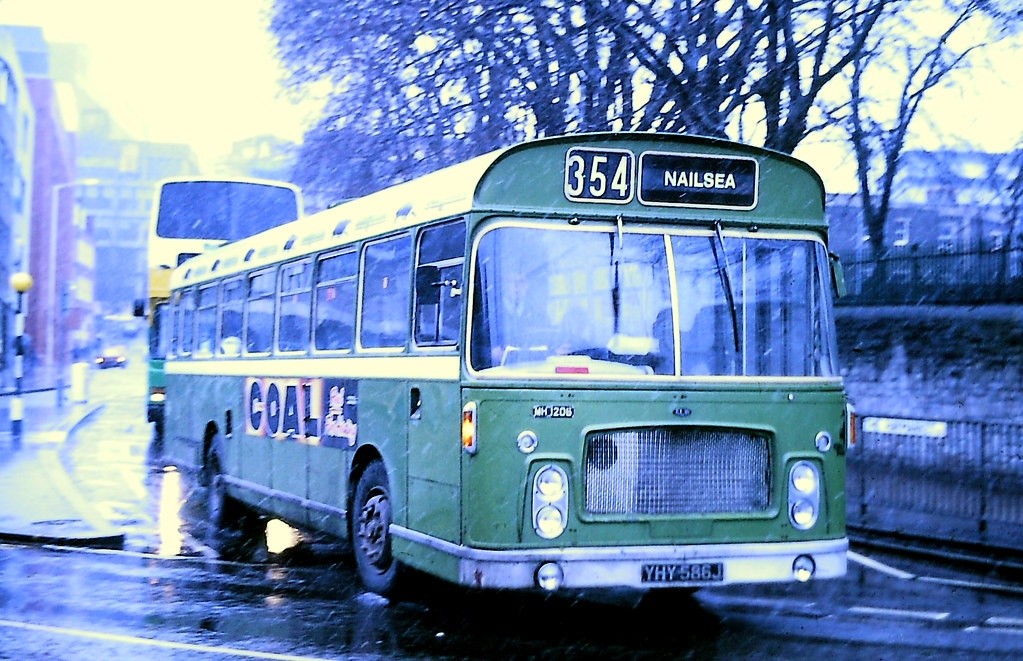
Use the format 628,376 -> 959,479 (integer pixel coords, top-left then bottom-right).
45,176 -> 102,378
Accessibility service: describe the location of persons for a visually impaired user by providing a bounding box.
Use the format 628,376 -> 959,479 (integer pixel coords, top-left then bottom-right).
210,300 -> 360,352
474,272 -> 569,366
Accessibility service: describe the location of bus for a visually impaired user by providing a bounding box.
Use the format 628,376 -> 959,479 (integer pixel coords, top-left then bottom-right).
155,128 -> 859,598
130,175 -> 305,425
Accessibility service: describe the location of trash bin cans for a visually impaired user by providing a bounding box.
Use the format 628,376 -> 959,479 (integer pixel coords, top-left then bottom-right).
69,363 -> 89,404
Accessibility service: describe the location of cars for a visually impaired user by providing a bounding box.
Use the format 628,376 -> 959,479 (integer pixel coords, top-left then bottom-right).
94,348 -> 129,370
